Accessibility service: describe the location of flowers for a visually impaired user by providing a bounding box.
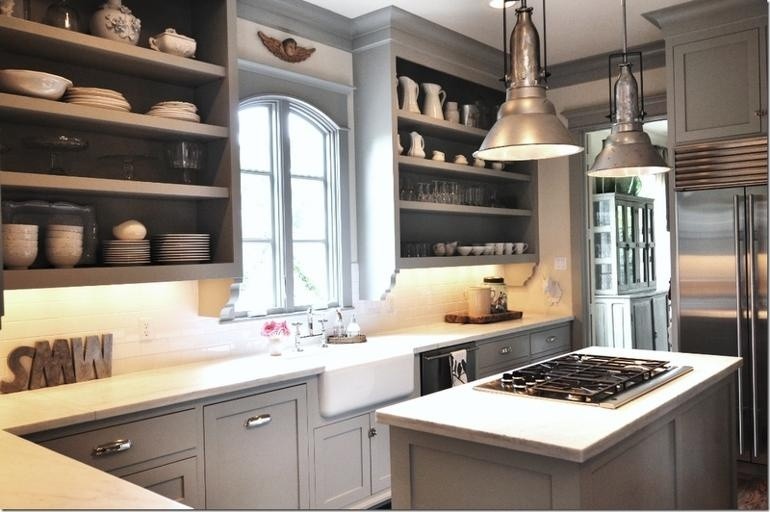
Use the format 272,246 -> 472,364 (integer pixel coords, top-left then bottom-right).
262,320 -> 291,340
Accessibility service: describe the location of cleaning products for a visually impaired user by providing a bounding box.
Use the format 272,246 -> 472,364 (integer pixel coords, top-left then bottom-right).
333,308 -> 346,337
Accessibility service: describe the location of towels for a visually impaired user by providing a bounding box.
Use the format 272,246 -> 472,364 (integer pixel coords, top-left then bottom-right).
449,350 -> 471,385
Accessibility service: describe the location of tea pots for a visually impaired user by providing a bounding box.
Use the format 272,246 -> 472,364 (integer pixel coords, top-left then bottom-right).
398,76 -> 422,114
431,149 -> 505,171
421,82 -> 450,121
432,242 -> 446,256
407,131 -> 426,158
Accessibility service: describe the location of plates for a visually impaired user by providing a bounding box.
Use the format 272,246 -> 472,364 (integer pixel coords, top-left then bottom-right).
152,233 -> 210,265
140,100 -> 204,122
102,238 -> 151,265
64,85 -> 133,113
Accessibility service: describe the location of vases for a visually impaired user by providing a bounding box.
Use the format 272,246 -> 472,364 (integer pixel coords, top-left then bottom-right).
270,341 -> 282,355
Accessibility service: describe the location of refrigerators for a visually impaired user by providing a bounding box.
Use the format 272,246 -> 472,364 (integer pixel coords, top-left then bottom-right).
666,134 -> 769,511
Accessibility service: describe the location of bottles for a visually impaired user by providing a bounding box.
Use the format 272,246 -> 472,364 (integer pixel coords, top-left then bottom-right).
461,104 -> 480,129
481,276 -> 507,314
445,100 -> 459,124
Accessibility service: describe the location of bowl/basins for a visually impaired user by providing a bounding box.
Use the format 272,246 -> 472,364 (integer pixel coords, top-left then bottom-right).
45,224 -> 84,268
1,68 -> 75,100
1,223 -> 39,270
456,246 -> 486,256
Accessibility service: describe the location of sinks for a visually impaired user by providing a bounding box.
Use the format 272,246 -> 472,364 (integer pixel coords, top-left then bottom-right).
318,350 -> 415,419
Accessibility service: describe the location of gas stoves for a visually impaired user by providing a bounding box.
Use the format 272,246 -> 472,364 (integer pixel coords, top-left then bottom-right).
469,354 -> 693,412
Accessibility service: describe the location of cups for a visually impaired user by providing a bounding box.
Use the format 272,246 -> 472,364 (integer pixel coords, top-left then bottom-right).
459,181 -> 503,208
400,186 -> 415,201
465,286 -> 496,317
485,242 -> 529,255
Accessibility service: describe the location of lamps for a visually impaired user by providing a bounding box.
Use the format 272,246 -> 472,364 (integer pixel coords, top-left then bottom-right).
470,1 -> 585,165
575,1 -> 680,178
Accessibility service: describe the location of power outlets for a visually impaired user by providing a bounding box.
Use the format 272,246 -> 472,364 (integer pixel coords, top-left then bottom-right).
138,316 -> 154,342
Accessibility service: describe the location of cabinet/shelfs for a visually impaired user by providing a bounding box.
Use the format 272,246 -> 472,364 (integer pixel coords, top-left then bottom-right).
474,319 -> 574,377
27,408 -> 200,510
595,296 -> 667,353
0,0 -> 244,290
352,40 -> 543,305
310,412 -> 391,508
587,195 -> 658,293
200,380 -> 309,509
645,1 -> 767,141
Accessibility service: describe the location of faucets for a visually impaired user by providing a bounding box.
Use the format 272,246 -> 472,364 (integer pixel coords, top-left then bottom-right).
306,307 -> 315,335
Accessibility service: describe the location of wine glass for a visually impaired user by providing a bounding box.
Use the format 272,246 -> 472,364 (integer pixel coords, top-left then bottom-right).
100,151 -> 157,183
415,178 -> 460,206
22,133 -> 90,176
